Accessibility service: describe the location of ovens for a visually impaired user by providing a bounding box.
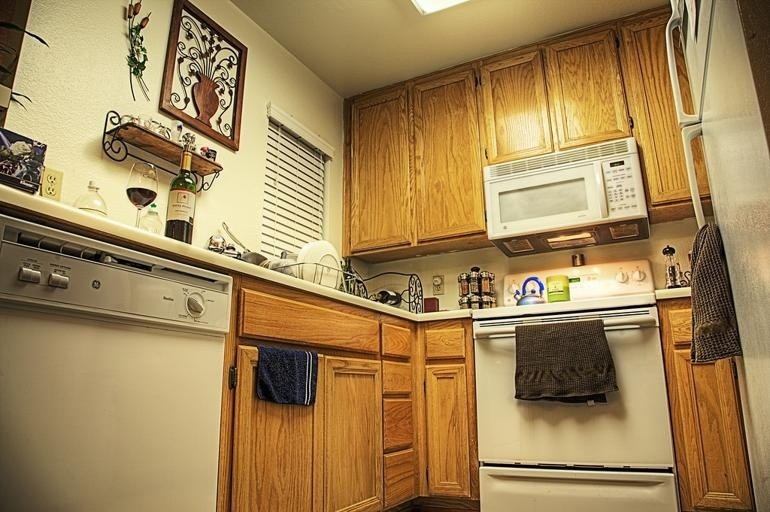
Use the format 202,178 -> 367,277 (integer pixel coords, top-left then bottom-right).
463,308 -> 684,512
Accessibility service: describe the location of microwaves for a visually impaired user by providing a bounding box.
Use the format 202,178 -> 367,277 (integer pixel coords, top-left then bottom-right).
484,137 -> 650,257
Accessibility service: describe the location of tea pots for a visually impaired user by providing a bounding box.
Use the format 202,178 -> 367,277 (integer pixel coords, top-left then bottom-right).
514,277 -> 546,305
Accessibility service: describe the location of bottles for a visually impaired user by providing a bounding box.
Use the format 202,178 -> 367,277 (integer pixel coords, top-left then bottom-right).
343,254 -> 354,293
371,290 -> 402,306
164,150 -> 196,247
73,180 -> 108,218
663,244 -> 683,289
458,272 -> 496,309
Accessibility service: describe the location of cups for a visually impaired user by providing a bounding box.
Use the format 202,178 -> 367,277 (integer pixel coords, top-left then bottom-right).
546,275 -> 570,301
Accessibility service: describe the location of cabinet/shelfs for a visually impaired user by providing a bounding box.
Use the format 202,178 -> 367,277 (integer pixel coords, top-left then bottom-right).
376,305 -> 421,512
342,63 -> 487,264
218,262 -> 382,512
478,20 -> 635,165
420,320 -> 481,512
616,4 -> 713,225
661,300 -> 756,512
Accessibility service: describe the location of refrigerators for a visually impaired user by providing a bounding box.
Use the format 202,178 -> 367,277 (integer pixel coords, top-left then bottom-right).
663,2 -> 770,512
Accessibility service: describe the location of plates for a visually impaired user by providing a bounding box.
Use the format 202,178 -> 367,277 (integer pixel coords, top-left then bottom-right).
297,240 -> 341,290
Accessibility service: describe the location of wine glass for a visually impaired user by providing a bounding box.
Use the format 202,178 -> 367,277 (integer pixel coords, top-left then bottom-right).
126,161 -> 158,229
